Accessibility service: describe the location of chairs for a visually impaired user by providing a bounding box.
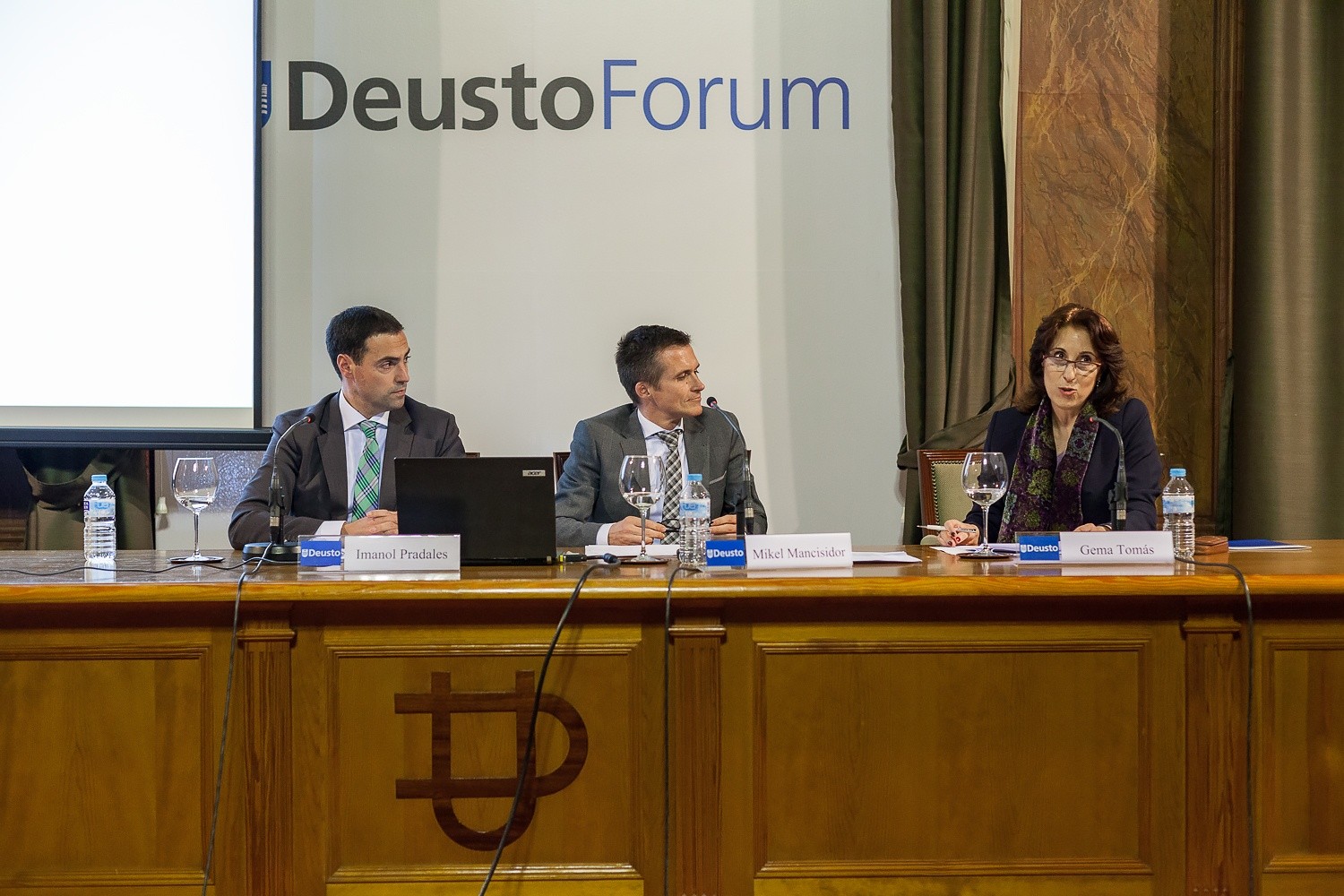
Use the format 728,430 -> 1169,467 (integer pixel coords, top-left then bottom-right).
920,449 -> 994,540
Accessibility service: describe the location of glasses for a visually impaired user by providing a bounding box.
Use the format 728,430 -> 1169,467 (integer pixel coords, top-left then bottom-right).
656,518 -> 680,533
1041,355 -> 1101,376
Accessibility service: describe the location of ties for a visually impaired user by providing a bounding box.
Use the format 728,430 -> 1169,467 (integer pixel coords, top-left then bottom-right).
351,419 -> 380,522
657,429 -> 683,545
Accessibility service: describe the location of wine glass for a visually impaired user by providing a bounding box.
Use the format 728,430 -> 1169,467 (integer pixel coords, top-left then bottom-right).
619,455 -> 666,564
959,451 -> 1010,557
168,457 -> 219,561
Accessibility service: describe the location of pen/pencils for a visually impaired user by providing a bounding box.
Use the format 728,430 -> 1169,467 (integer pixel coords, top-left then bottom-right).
916,524 -> 977,534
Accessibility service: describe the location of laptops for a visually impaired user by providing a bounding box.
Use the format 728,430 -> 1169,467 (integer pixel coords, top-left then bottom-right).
394,457 -> 566,566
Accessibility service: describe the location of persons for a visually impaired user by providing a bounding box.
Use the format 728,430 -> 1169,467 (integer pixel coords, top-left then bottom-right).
227,305 -> 466,551
935,303 -> 1164,548
552,325 -> 767,547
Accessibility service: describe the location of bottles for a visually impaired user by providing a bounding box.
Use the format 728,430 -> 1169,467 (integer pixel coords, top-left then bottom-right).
82,475 -> 117,561
1161,468 -> 1197,560
678,474 -> 712,564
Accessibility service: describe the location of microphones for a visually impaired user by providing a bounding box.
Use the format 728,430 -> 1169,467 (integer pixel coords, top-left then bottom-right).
707,396 -> 754,535
269,414 -> 316,541
1084,412 -> 1126,531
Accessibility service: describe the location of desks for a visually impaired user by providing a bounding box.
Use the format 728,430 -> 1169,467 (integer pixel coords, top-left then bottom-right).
0,544 -> 1344,896
